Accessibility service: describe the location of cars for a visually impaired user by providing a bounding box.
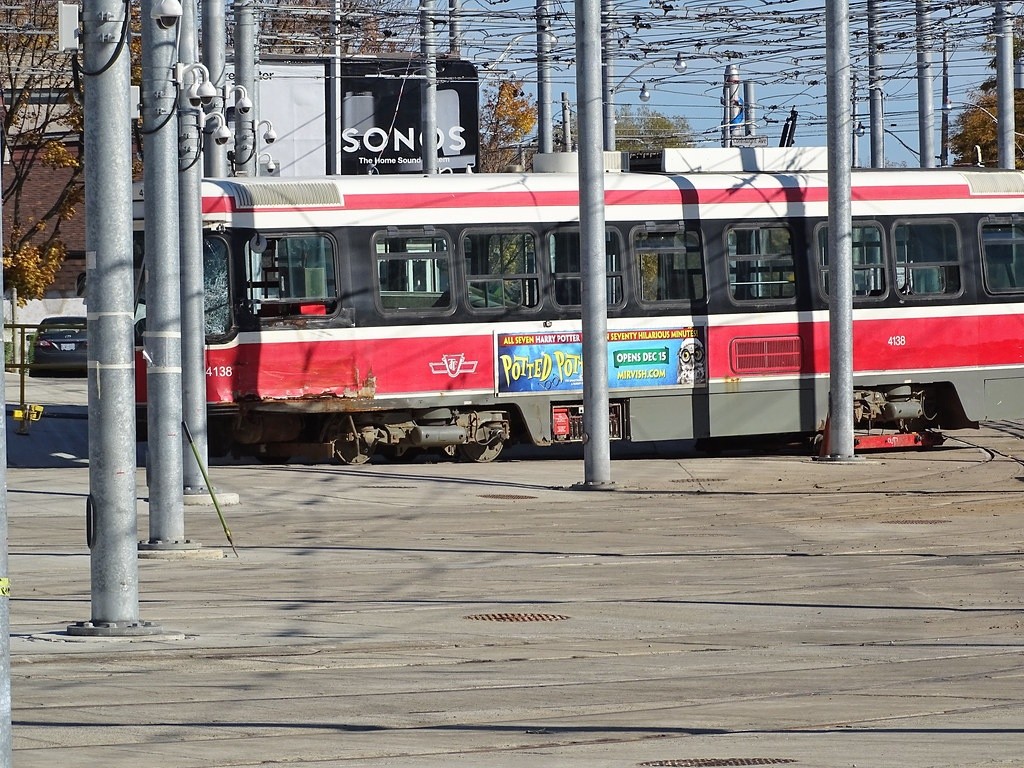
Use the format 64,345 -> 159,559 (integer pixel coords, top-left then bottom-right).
26,316 -> 88,377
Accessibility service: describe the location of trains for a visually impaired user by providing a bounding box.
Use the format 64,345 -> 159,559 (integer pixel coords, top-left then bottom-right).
134,146 -> 1023,467
